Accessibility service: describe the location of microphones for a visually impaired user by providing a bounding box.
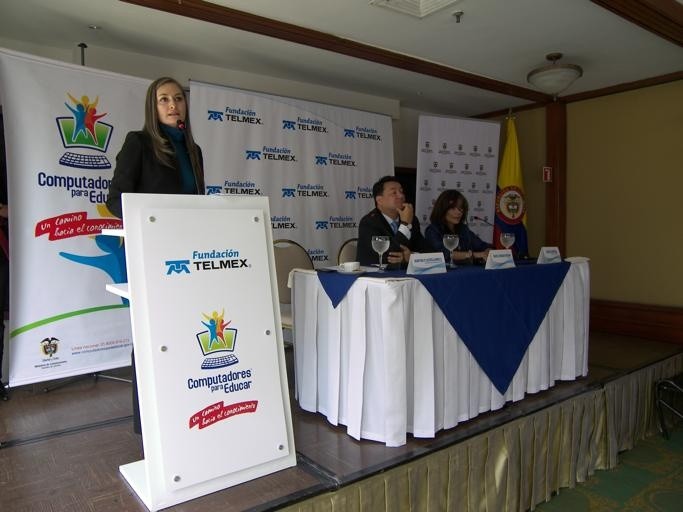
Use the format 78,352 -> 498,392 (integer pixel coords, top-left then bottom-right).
474,216 -> 538,265
176,119 -> 202,195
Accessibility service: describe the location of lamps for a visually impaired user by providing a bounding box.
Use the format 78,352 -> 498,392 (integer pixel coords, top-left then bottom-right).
525,49 -> 584,104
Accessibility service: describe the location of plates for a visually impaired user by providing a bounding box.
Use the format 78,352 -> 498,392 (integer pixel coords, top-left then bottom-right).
563,256 -> 591,263
335,268 -> 365,275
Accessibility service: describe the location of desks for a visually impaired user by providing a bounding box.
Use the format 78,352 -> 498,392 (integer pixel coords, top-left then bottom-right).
288,252 -> 590,450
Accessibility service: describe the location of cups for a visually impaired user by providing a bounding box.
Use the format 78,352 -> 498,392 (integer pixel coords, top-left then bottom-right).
338,261 -> 361,272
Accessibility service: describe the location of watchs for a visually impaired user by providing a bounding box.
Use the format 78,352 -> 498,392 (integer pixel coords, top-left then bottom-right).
400,221 -> 412,231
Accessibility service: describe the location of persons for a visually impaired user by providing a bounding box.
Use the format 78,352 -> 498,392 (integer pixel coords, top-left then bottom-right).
355,175 -> 436,270
423,189 -> 495,263
105,76 -> 206,435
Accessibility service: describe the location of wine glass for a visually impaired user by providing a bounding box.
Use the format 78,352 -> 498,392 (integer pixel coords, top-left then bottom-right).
442,234 -> 459,269
370,236 -> 389,273
499,232 -> 516,249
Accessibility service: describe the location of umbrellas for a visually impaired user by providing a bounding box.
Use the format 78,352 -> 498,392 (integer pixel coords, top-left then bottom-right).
492,112 -> 527,259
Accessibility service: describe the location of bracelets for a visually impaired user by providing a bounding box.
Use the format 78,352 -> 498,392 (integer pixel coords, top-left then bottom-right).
468,251 -> 473,256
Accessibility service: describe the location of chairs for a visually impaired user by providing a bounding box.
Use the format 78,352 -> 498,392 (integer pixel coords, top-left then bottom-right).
656,371 -> 683,440
272,240 -> 315,351
339,237 -> 359,266
